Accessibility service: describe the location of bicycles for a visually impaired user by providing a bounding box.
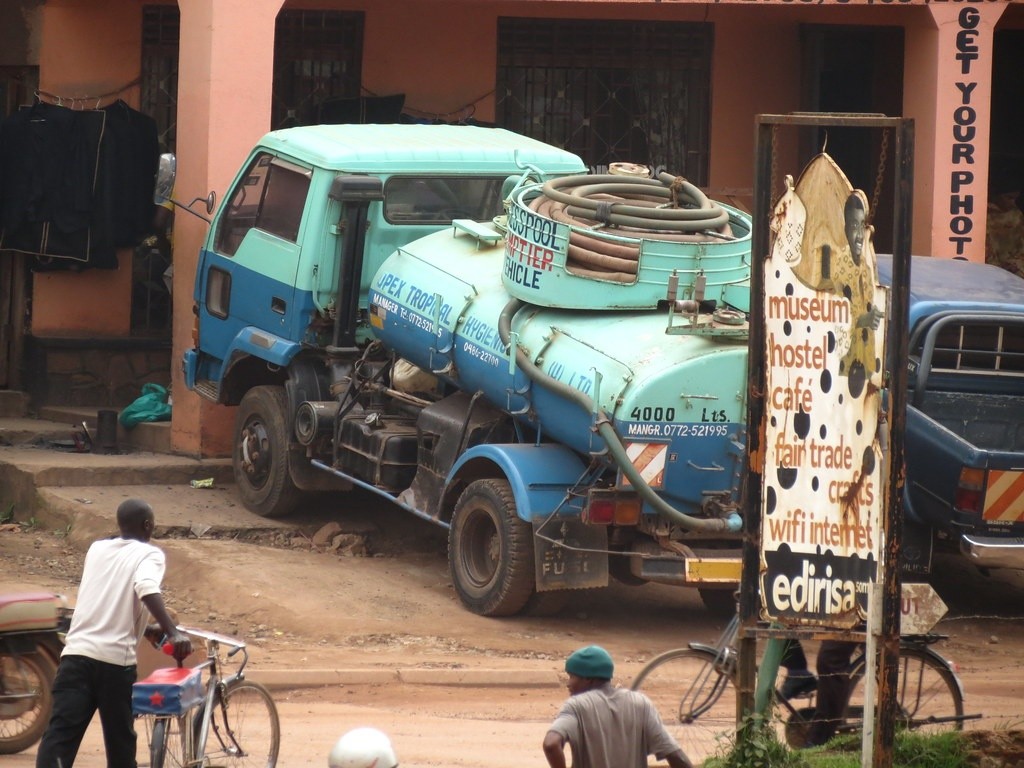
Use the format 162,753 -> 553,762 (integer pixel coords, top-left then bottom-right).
135,624 -> 282,768
629,585 -> 986,768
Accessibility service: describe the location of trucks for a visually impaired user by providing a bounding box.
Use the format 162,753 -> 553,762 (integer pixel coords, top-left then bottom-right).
150,122 -> 755,620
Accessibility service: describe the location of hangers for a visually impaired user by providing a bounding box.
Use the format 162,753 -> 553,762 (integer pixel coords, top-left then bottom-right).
433,104 -> 476,126
18,89 -> 101,123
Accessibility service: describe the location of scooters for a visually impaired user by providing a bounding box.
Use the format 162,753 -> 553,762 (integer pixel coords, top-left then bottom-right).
0,587 -> 77,755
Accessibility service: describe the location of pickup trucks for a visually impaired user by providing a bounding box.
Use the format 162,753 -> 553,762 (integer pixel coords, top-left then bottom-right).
873,254 -> 1023,599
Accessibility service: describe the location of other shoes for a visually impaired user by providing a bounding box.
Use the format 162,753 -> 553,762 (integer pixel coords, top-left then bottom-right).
772,672 -> 817,705
801,741 -> 817,750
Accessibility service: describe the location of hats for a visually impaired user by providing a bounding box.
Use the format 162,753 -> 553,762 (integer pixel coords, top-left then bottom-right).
565,645 -> 613,678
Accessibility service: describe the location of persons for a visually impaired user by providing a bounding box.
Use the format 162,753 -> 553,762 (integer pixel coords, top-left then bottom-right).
834,194 -> 885,379
775,627 -> 858,747
35,497 -> 194,768
542,645 -> 695,768
